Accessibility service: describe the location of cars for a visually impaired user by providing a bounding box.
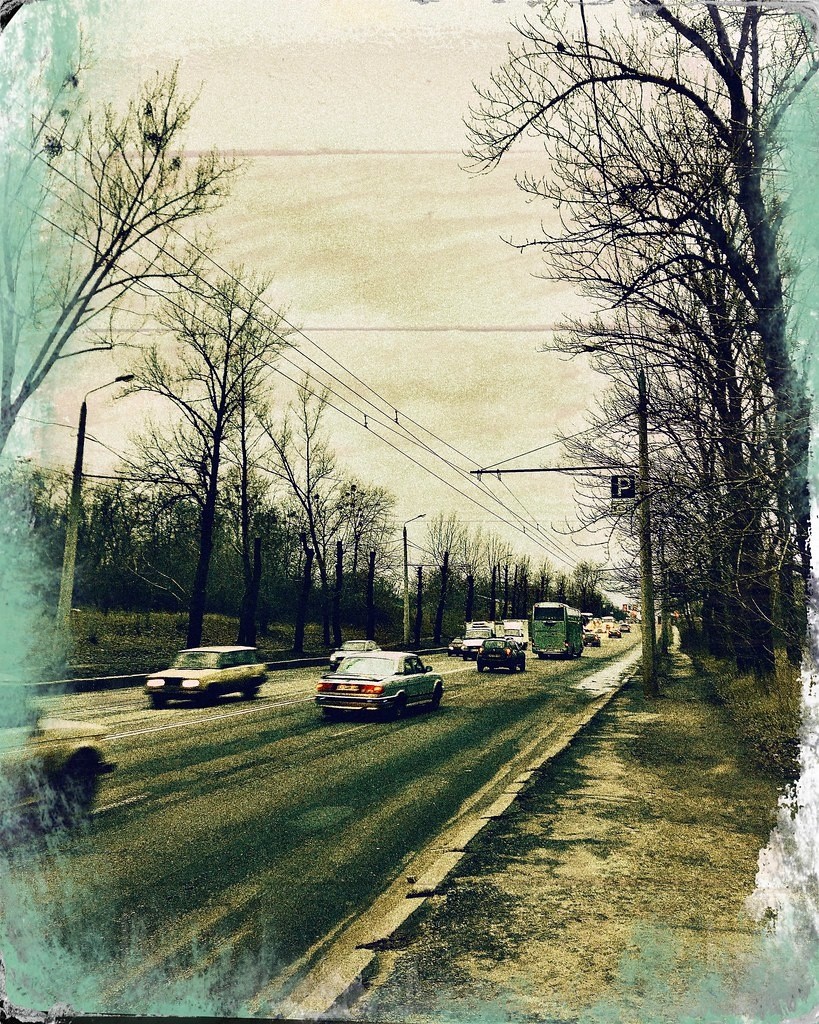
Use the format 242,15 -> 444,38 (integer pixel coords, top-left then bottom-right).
315,651 -> 444,716
329,639 -> 381,668
607,630 -> 622,638
447,635 -> 464,657
143,645 -> 269,705
583,634 -> 602,647
618,623 -> 632,633
1,673 -> 119,820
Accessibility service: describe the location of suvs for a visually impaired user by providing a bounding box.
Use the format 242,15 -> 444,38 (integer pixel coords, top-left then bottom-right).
475,636 -> 527,673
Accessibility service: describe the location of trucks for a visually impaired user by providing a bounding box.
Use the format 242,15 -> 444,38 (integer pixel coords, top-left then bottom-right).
461,619 -> 506,662
501,617 -> 529,651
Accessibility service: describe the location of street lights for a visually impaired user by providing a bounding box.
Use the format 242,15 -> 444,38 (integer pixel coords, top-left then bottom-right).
55,371 -> 136,663
403,511 -> 429,644
498,553 -> 512,606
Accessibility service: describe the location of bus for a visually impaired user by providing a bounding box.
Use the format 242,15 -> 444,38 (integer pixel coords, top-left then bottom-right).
531,602 -> 583,658
